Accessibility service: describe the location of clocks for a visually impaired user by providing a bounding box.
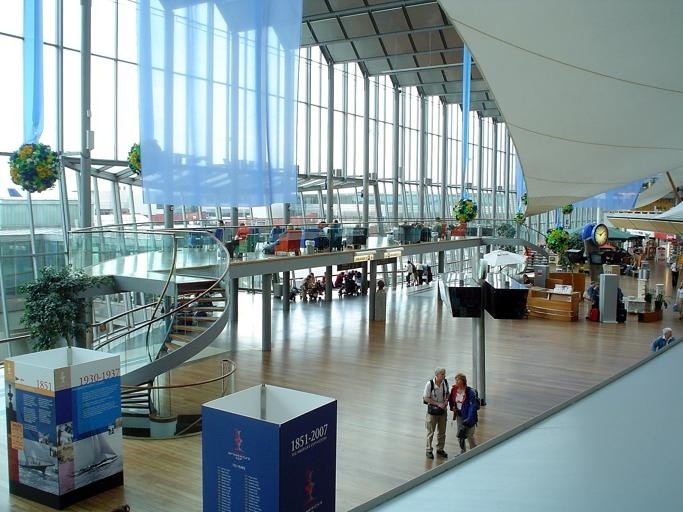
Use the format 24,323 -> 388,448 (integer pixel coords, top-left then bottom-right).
591,223 -> 608,246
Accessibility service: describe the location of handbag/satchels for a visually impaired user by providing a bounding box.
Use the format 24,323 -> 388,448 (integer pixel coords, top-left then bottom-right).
430,406 -> 444,415
465,387 -> 481,410
672,304 -> 681,312
583,291 -> 591,301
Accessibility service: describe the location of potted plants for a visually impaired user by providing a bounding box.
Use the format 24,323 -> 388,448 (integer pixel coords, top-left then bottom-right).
654,294 -> 672,311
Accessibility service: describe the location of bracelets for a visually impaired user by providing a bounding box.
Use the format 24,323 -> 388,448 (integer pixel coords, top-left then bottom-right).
446,399 -> 448,401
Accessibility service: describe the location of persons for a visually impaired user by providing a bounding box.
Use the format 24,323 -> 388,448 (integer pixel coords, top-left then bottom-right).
424,367 -> 450,459
586,255 -> 683,321
449,373 -> 478,454
214,219 -> 442,301
523,274 -> 530,284
651,328 -> 676,352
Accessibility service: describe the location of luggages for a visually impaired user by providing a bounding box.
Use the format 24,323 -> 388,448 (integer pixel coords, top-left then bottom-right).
617,302 -> 626,323
588,302 -> 599,322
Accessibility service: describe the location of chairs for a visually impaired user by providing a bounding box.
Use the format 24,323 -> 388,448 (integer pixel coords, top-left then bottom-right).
188,227 -> 367,256
386,222 -> 447,245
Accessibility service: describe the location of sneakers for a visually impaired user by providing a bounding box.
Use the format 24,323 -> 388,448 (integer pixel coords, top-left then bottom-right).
437,451 -> 447,458
426,452 -> 434,459
